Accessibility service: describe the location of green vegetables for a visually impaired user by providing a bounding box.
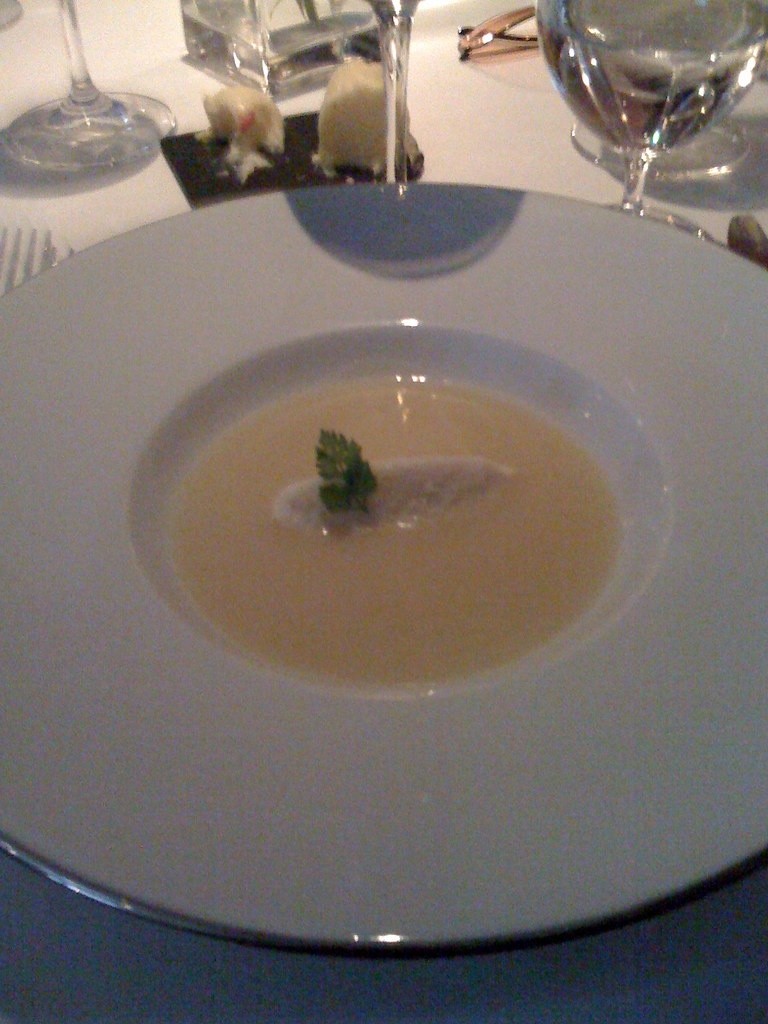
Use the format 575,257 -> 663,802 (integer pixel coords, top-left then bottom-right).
315,429 -> 377,516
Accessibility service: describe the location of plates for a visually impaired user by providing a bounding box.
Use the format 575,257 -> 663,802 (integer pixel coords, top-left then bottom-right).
160,110 -> 425,209
0,178 -> 768,954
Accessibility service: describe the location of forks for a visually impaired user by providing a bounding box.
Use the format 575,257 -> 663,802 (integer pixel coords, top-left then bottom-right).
0,227 -> 52,297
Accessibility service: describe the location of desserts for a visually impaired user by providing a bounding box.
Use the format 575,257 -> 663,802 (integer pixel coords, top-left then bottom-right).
318,61 -> 409,173
203,86 -> 284,180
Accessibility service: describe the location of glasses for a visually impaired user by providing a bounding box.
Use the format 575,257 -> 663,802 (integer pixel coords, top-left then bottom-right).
458,5 -> 538,61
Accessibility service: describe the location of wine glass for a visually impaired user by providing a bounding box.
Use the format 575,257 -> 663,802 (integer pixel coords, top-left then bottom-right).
0,0 -> 180,185
348,0 -> 429,184
526,0 -> 768,245
